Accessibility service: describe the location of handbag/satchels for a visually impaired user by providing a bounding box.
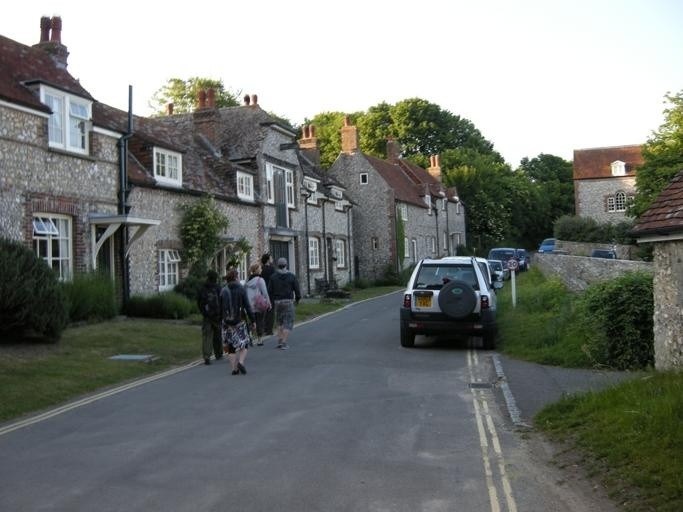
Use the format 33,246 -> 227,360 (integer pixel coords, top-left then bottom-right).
224,304 -> 241,325
255,294 -> 270,313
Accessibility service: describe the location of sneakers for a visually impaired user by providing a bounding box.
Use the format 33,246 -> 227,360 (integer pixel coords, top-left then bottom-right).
204,358 -> 210,365
276,338 -> 290,349
232,362 -> 246,375
215,355 -> 223,360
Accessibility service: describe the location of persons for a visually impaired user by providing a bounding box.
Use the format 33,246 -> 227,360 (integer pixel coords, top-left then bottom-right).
243,262 -> 272,346
219,270 -> 256,374
258,252 -> 277,334
194,269 -> 223,364
267,257 -> 300,349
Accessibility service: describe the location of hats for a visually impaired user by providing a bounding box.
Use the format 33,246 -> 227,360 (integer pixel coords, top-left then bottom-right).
277,257 -> 287,265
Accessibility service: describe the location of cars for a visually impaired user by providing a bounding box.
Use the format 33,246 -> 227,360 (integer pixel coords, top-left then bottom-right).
441,248 -> 527,287
537,237 -> 557,253
591,248 -> 615,259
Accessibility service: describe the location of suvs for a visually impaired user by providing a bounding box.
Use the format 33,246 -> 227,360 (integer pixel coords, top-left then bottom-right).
400,256 -> 498,349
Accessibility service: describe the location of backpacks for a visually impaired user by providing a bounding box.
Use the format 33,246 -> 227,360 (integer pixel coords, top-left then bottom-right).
200,287 -> 220,319
